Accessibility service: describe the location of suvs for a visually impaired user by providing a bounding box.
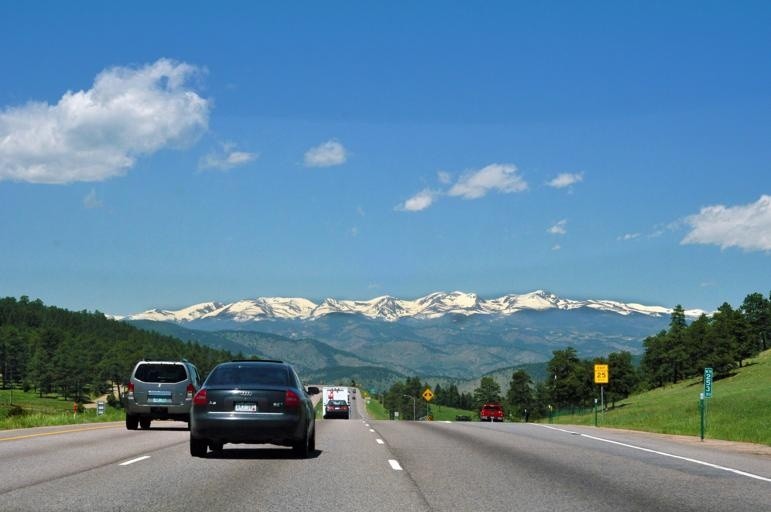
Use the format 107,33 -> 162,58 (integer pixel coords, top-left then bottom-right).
124,358 -> 201,430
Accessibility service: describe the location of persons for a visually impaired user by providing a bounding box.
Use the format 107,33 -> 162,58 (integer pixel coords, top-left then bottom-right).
521,408 -> 530,423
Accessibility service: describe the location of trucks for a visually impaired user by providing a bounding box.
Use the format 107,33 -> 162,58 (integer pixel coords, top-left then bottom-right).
323,387 -> 351,417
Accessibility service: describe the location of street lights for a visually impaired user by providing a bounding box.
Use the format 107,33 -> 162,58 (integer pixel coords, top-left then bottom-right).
404,394 -> 416,421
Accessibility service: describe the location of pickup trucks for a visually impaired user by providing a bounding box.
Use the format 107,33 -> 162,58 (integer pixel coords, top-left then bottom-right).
480,404 -> 503,421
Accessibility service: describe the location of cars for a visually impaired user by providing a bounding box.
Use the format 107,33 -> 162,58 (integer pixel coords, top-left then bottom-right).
189,359 -> 320,457
324,400 -> 350,419
